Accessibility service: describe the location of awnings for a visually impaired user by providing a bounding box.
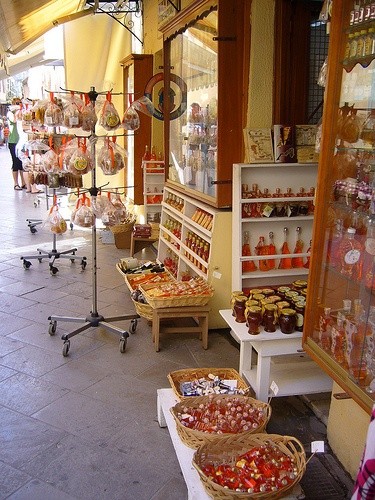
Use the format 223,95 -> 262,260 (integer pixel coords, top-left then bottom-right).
187,9 -> 218,54
0,24 -> 65,81
0,0 -> 101,77
168,32 -> 219,95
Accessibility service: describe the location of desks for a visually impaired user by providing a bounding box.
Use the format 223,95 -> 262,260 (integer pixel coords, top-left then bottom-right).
217,304 -> 335,412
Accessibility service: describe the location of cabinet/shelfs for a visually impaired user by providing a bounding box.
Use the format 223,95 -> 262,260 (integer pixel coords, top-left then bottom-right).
141,160 -> 165,225
233,165 -> 312,293
301,0 -> 375,412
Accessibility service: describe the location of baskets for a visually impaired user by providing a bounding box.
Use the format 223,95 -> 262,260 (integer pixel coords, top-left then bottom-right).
108,220 -> 137,234
116,260 -> 166,278
130,290 -> 154,321
167,367 -> 251,400
167,394 -> 271,449
191,432 -> 307,500
137,278 -> 214,309
125,272 -> 178,291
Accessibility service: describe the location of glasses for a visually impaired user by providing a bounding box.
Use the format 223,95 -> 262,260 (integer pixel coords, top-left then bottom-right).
15,101 -> 23,105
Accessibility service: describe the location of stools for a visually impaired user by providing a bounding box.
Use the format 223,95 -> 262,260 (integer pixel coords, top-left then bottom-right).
149,301 -> 210,351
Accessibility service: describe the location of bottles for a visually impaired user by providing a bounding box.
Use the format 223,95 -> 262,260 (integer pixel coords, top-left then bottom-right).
309,0 -> 375,405
174,394 -> 299,492
240,183 -> 313,273
119,190 -> 216,302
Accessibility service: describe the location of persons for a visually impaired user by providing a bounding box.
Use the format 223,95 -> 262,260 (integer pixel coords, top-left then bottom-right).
0,114 -> 8,148
6,98 -> 28,191
14,98 -> 45,193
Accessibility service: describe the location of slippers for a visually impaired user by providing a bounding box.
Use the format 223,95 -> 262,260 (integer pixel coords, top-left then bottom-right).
14,183 -> 29,190
26,188 -> 45,194
